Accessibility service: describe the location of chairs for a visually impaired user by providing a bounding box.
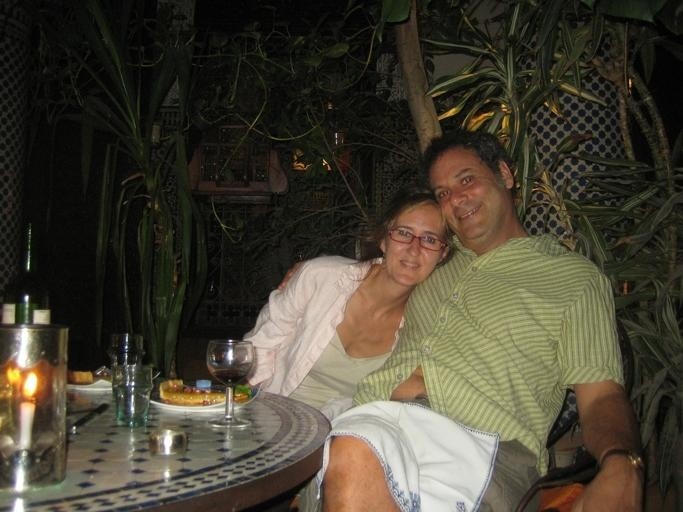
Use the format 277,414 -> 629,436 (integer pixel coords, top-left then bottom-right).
513,314 -> 635,512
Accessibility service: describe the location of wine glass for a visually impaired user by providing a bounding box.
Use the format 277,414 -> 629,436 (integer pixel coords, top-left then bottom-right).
115,334 -> 146,397
204,337 -> 256,430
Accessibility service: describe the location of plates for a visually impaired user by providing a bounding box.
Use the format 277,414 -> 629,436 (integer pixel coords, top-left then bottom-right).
151,381 -> 261,417
65,376 -> 113,395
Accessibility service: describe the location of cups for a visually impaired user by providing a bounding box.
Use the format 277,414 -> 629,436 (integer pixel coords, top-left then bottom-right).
0,323 -> 69,487
112,364 -> 153,428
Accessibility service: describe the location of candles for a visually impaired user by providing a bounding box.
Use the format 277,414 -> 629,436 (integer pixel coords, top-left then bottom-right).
20,402 -> 35,451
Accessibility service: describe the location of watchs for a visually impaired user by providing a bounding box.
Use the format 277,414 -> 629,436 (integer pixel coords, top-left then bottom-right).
601,449 -> 645,468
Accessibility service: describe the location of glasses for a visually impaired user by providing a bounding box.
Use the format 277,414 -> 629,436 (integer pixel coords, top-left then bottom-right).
387,223 -> 447,252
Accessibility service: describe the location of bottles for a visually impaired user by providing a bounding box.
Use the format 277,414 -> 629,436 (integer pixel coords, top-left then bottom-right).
12,219 -> 53,327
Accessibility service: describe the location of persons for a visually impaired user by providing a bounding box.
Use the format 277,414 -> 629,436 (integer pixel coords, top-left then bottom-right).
277,129 -> 648,512
232,188 -> 452,430
